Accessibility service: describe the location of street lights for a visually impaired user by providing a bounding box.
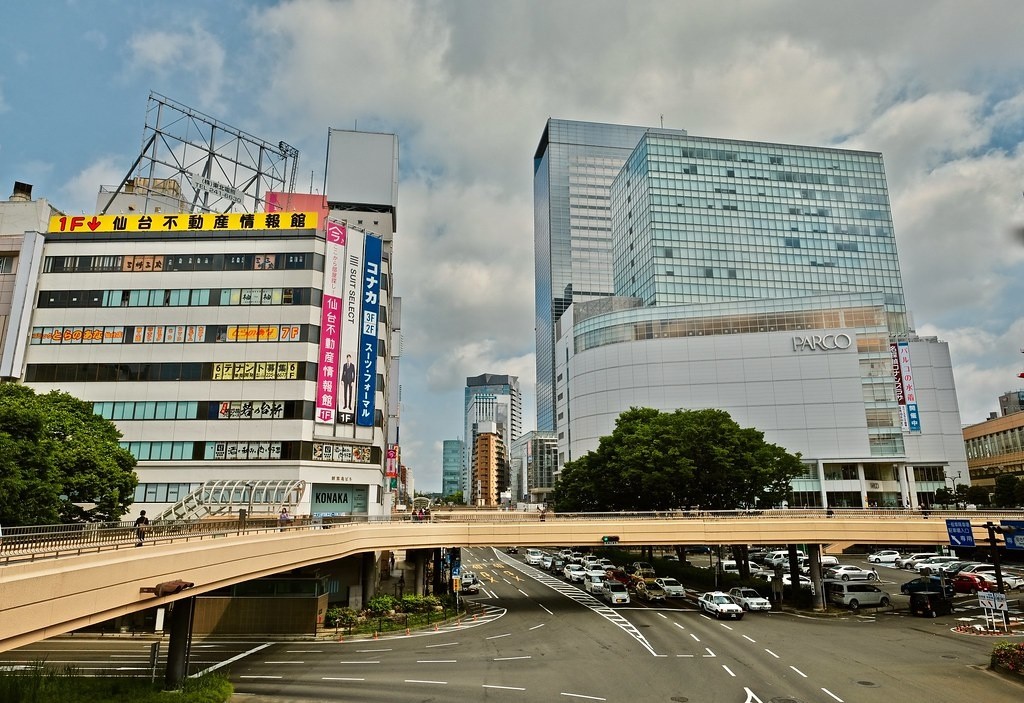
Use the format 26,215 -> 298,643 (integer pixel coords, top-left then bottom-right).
943,470 -> 962,510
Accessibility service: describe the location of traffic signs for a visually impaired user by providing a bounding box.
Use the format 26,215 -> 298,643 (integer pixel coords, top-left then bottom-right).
946,519 -> 976,548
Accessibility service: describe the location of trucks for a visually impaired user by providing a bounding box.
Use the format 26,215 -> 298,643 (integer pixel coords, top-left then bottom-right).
460,572 -> 480,594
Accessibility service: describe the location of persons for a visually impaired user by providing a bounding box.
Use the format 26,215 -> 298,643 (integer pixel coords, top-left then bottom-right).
130,510 -> 149,547
500,502 -> 547,523
278,508 -> 289,532
869,497 -> 947,520
411,507 -> 431,523
341,354 -> 356,410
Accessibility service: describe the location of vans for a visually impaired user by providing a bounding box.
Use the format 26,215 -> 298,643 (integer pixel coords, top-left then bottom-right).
764,549 -> 804,569
719,561 -> 764,575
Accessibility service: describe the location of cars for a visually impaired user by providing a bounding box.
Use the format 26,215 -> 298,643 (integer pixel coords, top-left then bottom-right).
524,548 -> 686,606
698,591 -> 743,621
935,561 -> 1024,594
827,564 -> 875,581
506,546 -> 519,554
753,556 -> 839,586
729,588 -> 772,613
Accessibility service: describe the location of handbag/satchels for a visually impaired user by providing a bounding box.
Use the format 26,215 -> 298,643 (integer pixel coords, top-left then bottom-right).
139,517 -> 148,530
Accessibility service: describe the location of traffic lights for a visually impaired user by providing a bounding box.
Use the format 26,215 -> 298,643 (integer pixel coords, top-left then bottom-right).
602,536 -> 619,542
990,523 -> 1016,535
155,579 -> 195,599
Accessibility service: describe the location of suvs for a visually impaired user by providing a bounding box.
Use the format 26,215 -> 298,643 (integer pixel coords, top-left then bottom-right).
901,577 -> 956,598
898,552 -> 938,570
830,581 -> 890,610
909,591 -> 954,618
868,550 -> 901,564
914,556 -> 959,575
